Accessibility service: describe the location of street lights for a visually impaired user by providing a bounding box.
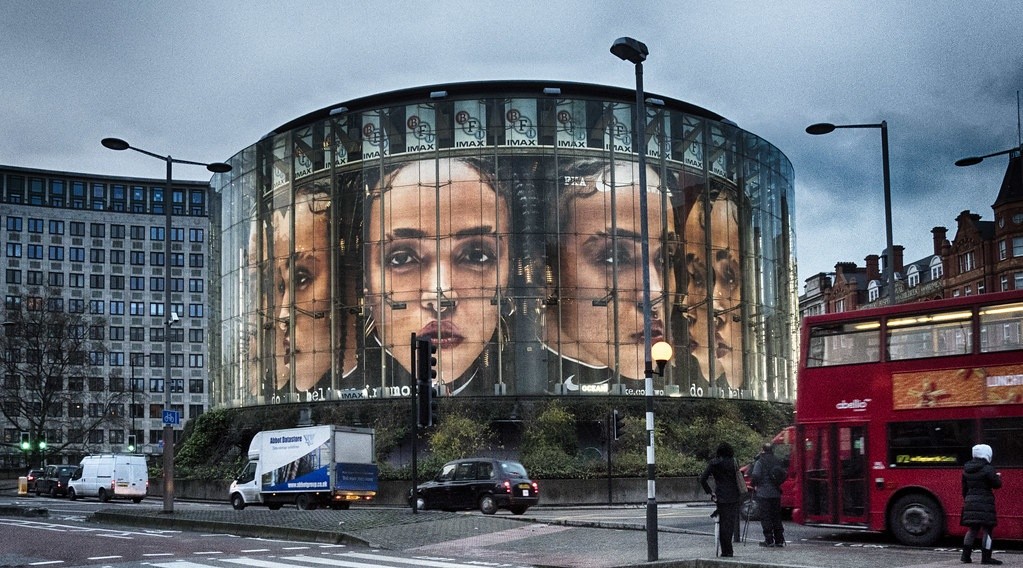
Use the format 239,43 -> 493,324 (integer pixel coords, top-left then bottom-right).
806,118 -> 898,304
609,35 -> 673,562
100,137 -> 234,504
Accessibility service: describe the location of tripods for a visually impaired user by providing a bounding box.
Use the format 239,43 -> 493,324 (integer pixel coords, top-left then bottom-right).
742,483 -> 787,546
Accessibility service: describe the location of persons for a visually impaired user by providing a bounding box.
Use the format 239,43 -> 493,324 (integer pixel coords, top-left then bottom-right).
245,155 -> 757,396
698,441 -> 741,558
749,442 -> 785,547
960,444 -> 1003,564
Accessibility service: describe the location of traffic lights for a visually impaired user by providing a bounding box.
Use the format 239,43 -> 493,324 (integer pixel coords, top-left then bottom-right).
417,334 -> 438,430
39,433 -> 46,451
20,432 -> 31,450
126,433 -> 136,453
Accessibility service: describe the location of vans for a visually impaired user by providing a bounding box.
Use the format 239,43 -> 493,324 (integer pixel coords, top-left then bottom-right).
67,453 -> 149,502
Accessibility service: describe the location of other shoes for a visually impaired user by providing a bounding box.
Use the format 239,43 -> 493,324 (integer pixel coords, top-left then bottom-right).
760,542 -> 774,547
721,552 -> 733,557
775,542 -> 783,547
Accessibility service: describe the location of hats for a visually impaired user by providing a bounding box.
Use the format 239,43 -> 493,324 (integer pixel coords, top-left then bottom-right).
972,444 -> 993,463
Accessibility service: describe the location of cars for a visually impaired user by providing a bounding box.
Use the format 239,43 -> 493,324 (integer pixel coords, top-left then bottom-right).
25,469 -> 42,493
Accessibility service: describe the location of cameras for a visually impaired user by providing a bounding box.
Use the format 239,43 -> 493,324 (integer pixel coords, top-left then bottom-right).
754,451 -> 762,460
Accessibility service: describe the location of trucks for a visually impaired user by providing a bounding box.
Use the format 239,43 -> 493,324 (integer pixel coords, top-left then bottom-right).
228,426 -> 379,511
734,425 -> 795,519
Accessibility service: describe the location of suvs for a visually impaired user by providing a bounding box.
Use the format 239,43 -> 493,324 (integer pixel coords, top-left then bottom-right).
33,464 -> 78,498
406,457 -> 540,515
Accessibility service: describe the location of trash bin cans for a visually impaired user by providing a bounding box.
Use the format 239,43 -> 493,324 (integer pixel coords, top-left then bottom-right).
18,476 -> 27,494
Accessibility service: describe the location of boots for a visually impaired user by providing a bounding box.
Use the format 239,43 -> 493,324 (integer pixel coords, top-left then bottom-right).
981,549 -> 1003,565
960,544 -> 973,562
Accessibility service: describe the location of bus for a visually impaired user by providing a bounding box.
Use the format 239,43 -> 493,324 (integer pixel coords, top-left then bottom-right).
795,285 -> 1023,545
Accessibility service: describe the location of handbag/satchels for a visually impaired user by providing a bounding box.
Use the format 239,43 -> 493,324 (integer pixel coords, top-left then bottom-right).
733,457 -> 748,494
